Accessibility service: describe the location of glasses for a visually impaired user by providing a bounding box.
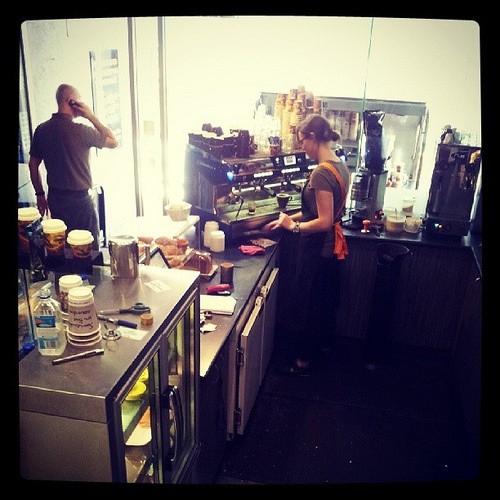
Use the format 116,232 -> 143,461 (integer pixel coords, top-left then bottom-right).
297,136 -> 308,146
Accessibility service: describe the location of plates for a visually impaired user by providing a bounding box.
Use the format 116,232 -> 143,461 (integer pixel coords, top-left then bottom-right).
124,366 -> 150,401
125,427 -> 152,446
181,264 -> 219,276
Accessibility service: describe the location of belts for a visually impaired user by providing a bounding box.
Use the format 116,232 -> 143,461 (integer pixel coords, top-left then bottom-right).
49,187 -> 89,197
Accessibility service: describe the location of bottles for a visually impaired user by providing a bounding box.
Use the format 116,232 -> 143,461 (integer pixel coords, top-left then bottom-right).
442,130 -> 454,145
33,288 -> 67,356
204,221 -> 225,253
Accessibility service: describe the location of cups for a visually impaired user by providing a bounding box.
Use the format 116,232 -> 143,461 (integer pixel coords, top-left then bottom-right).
108,235 -> 140,278
59,274 -> 102,346
188,86 -> 360,158
277,192 -> 293,208
40,219 -> 67,261
18,207 -> 44,254
248,201 -> 259,215
67,229 -> 97,262
221,263 -> 236,283
387,199 -> 422,234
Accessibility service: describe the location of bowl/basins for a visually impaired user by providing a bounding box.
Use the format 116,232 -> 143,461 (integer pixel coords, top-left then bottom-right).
164,203 -> 192,220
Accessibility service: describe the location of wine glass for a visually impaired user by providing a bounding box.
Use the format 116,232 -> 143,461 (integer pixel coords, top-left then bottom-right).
102,307 -> 121,341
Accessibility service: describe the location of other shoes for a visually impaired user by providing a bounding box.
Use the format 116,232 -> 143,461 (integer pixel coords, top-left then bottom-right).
274,360 -> 311,376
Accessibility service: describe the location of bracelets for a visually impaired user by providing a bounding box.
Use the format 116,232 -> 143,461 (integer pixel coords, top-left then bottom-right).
35,190 -> 45,196
293,221 -> 301,233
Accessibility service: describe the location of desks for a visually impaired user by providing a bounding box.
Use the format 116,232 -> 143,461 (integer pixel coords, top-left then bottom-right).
18,163 -> 48,208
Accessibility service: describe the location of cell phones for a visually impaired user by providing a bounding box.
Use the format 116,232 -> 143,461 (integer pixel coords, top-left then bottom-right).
68,99 -> 80,108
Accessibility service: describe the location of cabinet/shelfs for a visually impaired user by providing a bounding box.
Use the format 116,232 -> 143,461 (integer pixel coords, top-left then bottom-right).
336,238 -> 482,430
227,246 -> 282,441
254,84 -> 362,209
18,265 -> 201,484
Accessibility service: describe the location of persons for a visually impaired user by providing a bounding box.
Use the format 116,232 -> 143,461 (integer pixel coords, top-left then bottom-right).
29,83 -> 117,251
264,114 -> 351,377
